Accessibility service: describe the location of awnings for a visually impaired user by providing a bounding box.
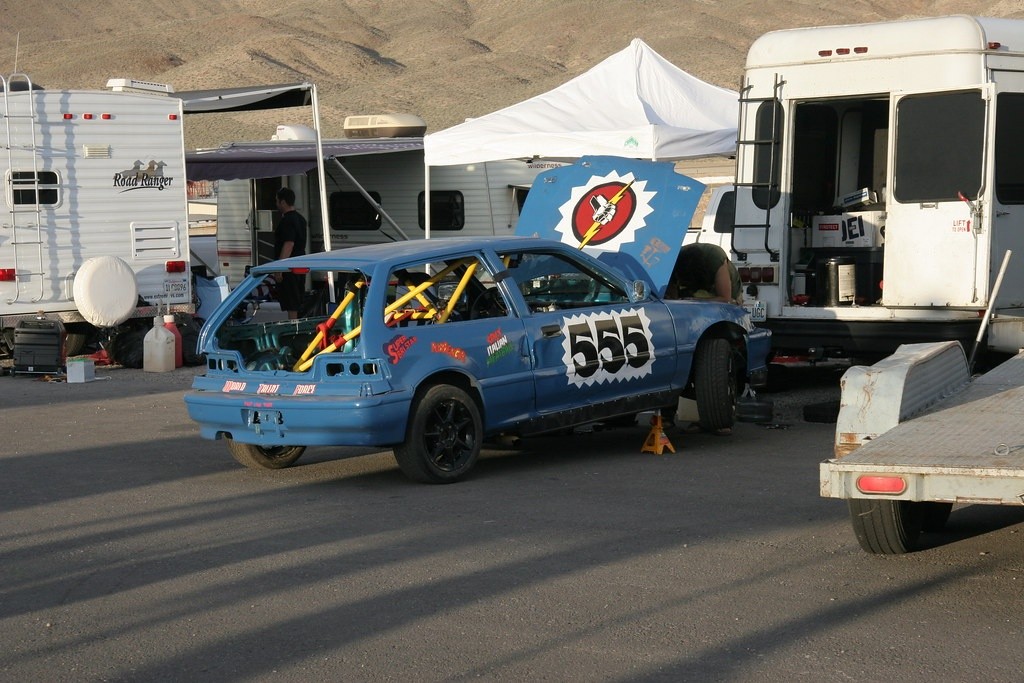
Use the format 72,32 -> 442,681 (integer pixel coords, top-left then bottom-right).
168,82 -> 335,306
186,137 -> 424,277
508,184 -> 532,228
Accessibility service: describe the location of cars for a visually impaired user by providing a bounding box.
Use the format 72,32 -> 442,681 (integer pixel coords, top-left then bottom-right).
184,154 -> 772,486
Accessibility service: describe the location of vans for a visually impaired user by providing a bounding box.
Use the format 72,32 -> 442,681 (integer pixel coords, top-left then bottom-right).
699,186 -> 734,263
730,14 -> 1024,366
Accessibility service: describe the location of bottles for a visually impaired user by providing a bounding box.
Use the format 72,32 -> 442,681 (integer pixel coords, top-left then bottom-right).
246,300 -> 255,318
142,315 -> 184,373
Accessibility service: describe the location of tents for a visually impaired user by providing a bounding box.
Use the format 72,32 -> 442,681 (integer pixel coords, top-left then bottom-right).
423,37 -> 742,276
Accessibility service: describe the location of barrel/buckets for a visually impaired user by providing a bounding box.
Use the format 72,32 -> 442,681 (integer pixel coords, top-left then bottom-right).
824,256 -> 859,306
731,399 -> 774,425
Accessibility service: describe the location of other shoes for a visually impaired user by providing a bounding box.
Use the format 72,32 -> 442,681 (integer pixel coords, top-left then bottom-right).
682,421 -> 704,433
714,427 -> 733,436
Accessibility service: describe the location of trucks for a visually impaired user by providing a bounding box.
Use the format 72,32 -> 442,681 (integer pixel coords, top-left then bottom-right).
0,73 -> 198,367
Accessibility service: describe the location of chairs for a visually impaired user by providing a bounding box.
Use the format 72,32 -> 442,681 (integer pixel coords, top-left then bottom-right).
394,272 -> 436,326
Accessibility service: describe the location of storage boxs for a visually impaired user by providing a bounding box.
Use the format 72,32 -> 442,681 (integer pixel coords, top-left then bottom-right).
65,360 -> 95,384
810,210 -> 886,249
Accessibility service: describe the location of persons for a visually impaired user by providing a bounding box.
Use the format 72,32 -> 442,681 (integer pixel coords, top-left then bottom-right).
273,187 -> 307,319
663,243 -> 744,437
